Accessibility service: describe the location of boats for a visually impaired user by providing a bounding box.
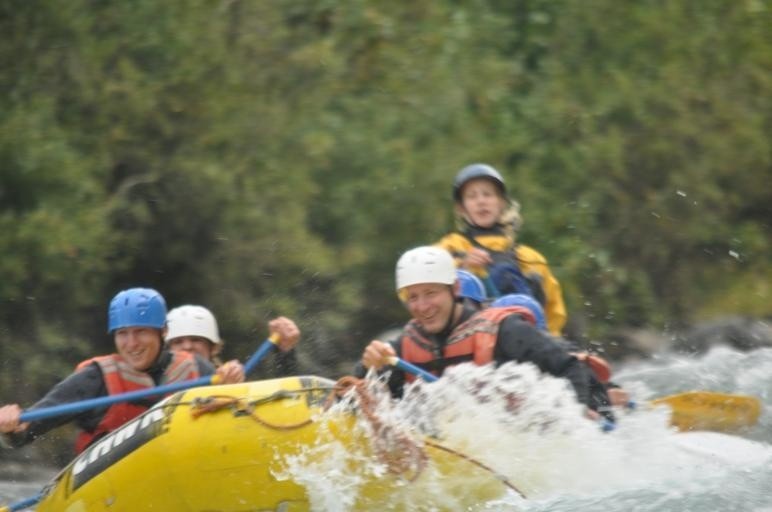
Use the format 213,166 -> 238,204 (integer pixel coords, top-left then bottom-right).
36,376 -> 764,512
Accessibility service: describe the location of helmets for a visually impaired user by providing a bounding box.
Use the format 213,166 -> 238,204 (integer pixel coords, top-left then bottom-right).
164,305 -> 220,343
396,246 -> 487,302
108,288 -> 166,333
491,294 -> 545,330
453,163 -> 505,195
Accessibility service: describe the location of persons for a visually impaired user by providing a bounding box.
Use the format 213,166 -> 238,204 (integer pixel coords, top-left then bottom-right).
163,305 -> 302,379
355,247 -> 616,423
397,163 -> 630,406
1,287 -> 246,455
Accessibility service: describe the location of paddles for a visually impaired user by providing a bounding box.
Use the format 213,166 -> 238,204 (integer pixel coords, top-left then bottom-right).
627,392 -> 761,433
